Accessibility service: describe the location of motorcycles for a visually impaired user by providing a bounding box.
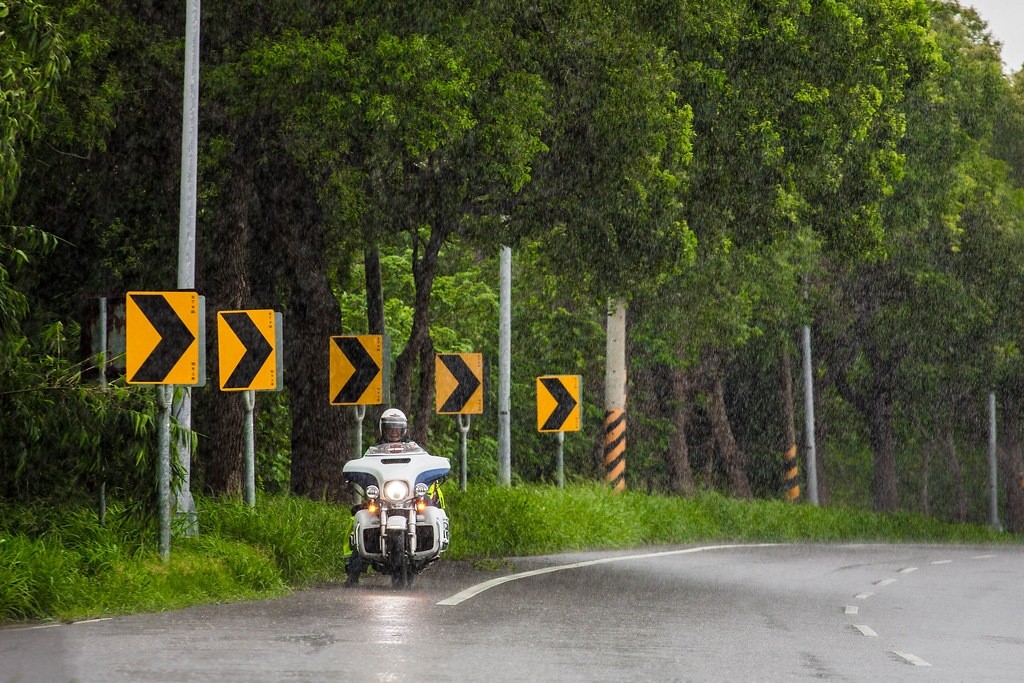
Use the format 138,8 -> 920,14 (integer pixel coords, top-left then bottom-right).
342,452 -> 451,587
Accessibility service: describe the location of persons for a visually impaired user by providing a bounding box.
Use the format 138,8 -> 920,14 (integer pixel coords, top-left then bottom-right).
343,410 -> 434,587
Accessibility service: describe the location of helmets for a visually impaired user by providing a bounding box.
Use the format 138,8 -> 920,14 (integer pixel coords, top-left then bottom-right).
379,408 -> 407,438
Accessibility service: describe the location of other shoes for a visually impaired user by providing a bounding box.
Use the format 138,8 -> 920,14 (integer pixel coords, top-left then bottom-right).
344,575 -> 359,588
